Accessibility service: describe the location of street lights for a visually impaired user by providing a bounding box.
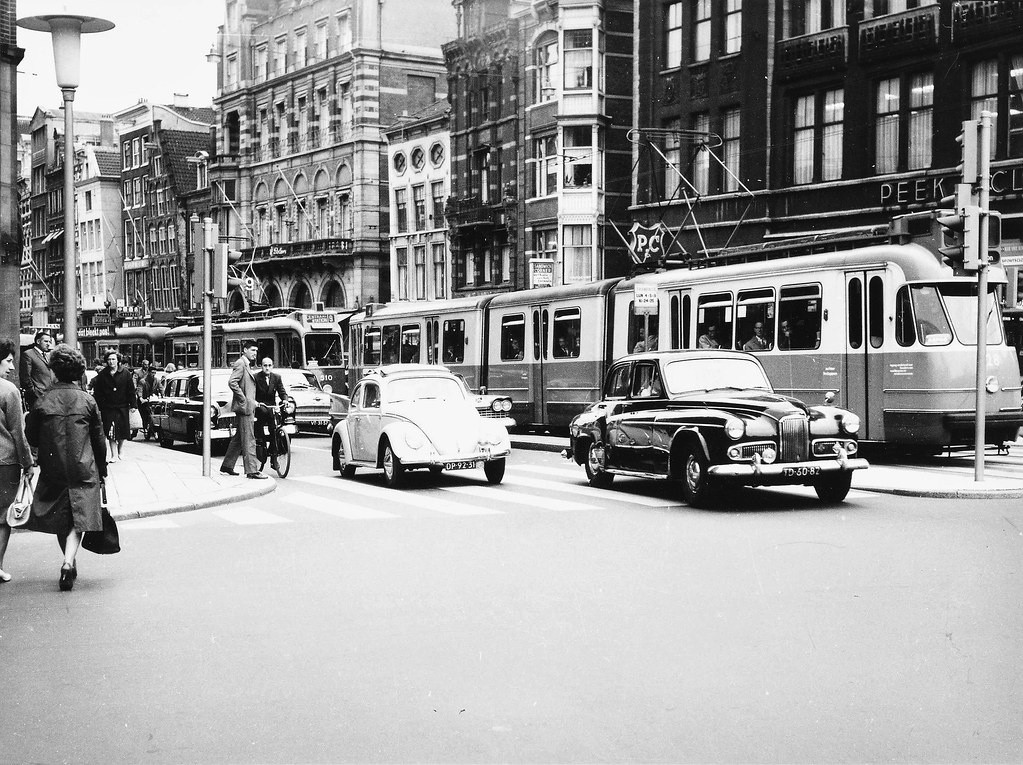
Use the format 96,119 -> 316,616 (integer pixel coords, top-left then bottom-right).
11,13 -> 117,353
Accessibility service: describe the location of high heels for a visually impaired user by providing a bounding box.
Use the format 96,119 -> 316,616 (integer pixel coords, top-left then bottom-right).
59,563 -> 73,591
72,559 -> 77,578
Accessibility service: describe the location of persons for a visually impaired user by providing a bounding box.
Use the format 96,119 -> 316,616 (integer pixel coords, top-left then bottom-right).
23,333 -> 57,411
370,320 -> 796,363
25,344 -> 107,590
88,352 -> 175,464
220,340 -> 269,479
640,373 -> 661,397
252,357 -> 290,470
0,339 -> 36,582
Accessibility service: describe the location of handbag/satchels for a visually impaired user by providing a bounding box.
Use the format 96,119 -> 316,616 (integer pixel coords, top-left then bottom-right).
81,484 -> 120,555
129,409 -> 143,429
6,474 -> 34,528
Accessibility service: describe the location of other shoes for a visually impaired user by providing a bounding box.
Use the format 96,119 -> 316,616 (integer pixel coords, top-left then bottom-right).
109,457 -> 116,463
0,569 -> 11,581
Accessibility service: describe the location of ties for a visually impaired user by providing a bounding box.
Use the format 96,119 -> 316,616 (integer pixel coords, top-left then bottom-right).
42,353 -> 47,362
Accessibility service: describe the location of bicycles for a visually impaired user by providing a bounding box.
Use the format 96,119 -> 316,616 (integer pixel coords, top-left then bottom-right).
254,399 -> 294,479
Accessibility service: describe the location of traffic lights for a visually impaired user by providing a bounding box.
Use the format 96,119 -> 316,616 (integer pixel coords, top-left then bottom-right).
211,242 -> 244,300
988,251 -> 1002,265
987,212 -> 1002,249
938,205 -> 980,279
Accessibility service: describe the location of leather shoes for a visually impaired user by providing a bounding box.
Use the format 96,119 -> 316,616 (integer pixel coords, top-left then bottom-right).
220,466 -> 239,475
247,473 -> 268,479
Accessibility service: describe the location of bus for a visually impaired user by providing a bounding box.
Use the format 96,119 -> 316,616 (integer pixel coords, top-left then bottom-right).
348,209 -> 1023,465
168,302 -> 366,396
78,325 -> 171,377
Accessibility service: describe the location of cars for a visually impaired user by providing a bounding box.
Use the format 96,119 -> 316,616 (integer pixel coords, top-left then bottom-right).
331,363 -> 512,488
557,348 -> 871,514
252,367 -> 334,435
149,367 -> 238,453
